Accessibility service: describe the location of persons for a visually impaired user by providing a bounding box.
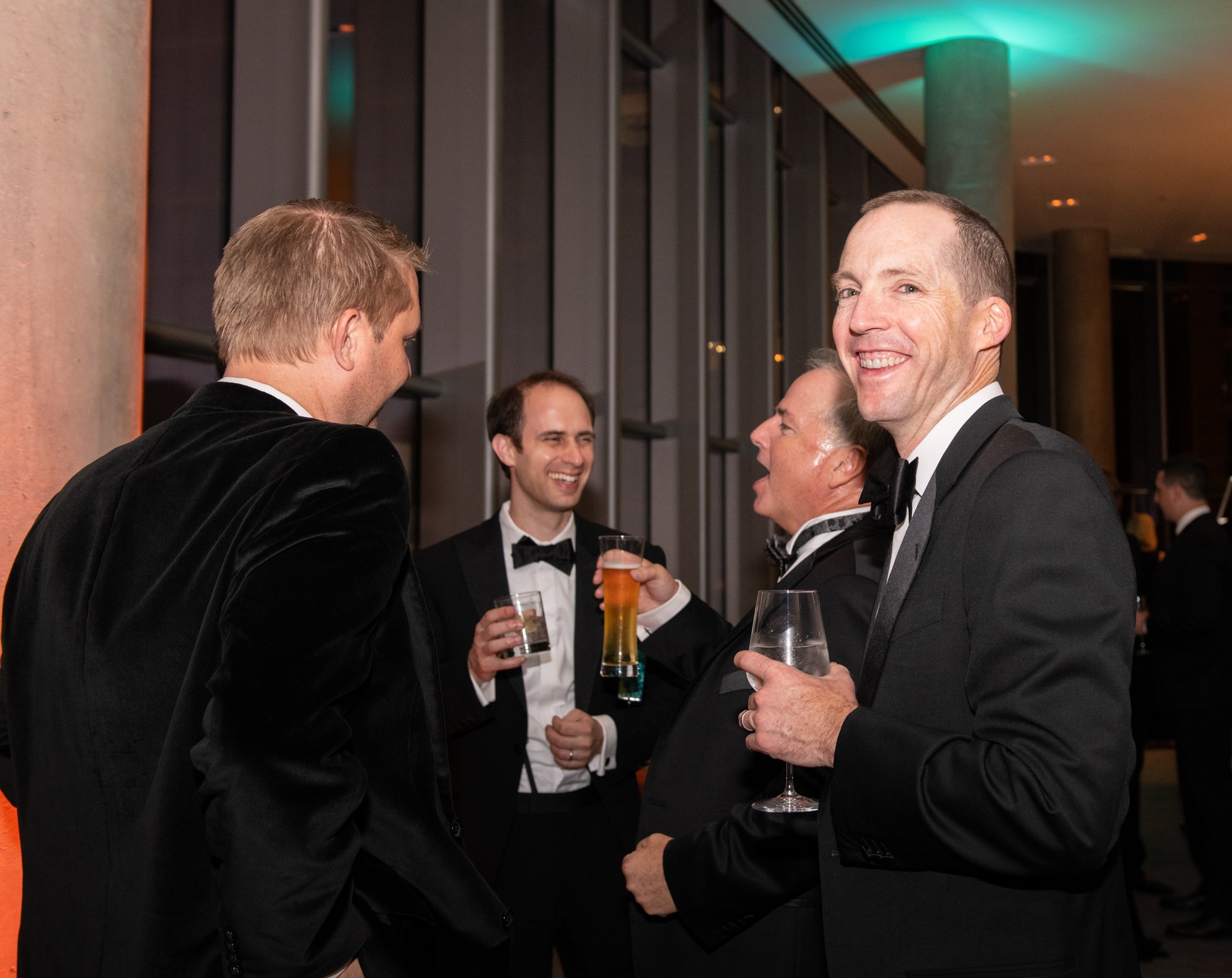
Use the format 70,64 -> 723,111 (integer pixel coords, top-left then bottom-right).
1134,459 -> 1232,950
592,346 -> 900,978
412,368 -> 691,977
2,196 -> 504,977
733,191 -> 1136,978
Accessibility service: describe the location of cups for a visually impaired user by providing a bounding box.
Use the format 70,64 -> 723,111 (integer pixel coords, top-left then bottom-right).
1133,596 -> 1153,655
599,535 -> 645,678
494,591 -> 551,659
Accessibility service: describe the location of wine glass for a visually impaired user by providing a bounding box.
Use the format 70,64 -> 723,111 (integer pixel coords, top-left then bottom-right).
745,589 -> 829,812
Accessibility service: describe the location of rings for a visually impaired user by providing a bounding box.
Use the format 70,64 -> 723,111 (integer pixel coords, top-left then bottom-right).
741,710 -> 753,731
569,749 -> 573,760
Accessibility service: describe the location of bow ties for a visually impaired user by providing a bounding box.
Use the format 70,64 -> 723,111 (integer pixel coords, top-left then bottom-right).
858,458 -> 918,525
511,535 -> 574,576
764,511 -> 870,576
